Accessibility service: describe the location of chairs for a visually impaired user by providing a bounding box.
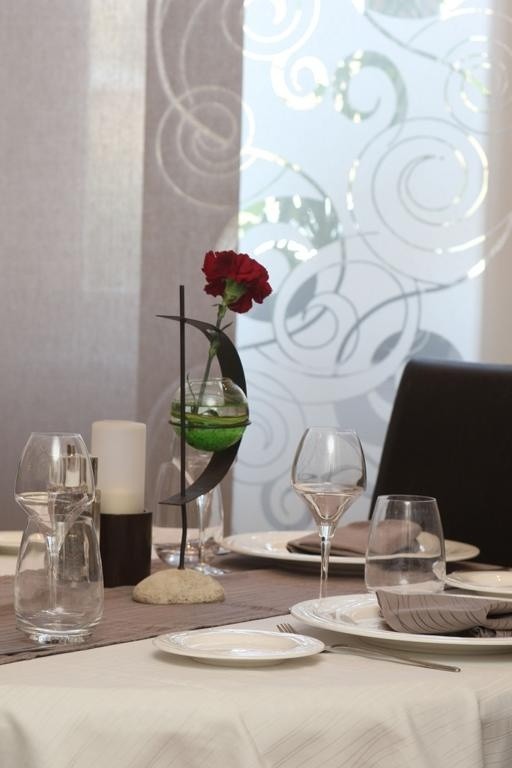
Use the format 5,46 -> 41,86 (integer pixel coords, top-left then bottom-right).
367,359 -> 512,569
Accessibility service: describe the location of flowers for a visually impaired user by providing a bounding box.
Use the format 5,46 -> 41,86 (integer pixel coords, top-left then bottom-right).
191,250 -> 272,415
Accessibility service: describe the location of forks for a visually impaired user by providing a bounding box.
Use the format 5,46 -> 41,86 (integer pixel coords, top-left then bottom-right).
275,621 -> 462,674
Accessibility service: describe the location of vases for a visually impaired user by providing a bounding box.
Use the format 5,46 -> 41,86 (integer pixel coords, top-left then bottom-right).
170,378 -> 248,449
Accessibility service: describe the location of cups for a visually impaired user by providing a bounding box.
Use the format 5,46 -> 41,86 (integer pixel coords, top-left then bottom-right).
94,510 -> 153,592
7,511 -> 107,649
362,491 -> 450,606
150,457 -> 227,566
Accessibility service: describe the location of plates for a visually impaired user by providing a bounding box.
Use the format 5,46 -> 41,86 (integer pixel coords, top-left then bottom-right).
288,590 -> 512,657
148,625 -> 328,669
440,569 -> 512,598
216,525 -> 484,578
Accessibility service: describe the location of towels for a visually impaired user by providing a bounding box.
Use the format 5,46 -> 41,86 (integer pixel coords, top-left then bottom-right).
285,521 -> 421,558
375,591 -> 512,638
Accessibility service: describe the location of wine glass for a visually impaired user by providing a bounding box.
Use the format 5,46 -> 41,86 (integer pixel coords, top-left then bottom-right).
171,423 -> 230,576
13,428 -> 97,645
282,424 -> 370,621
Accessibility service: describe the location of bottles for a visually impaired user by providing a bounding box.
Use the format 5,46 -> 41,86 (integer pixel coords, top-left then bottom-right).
44,442 -> 100,588
